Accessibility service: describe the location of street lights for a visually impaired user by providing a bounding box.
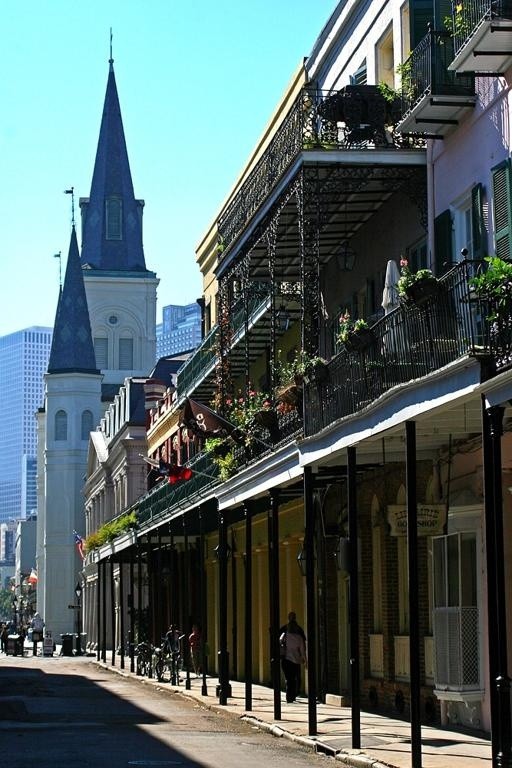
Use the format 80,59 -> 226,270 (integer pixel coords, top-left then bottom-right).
75,581 -> 82,655
13,595 -> 23,629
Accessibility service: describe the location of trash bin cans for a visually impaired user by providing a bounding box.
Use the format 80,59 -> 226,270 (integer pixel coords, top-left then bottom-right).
6,634 -> 23,656
60,634 -> 73,656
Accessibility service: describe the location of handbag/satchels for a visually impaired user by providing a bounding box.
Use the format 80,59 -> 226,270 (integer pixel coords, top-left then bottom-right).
280,643 -> 286,656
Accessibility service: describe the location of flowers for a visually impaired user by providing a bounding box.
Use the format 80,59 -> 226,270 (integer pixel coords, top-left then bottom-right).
336,310 -> 368,344
226,391 -> 269,422
399,257 -> 432,296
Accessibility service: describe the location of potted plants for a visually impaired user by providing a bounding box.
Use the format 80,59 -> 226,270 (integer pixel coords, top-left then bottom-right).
271,349 -> 327,403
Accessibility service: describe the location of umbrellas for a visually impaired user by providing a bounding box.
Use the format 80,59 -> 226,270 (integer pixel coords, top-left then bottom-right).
381,259 -> 410,355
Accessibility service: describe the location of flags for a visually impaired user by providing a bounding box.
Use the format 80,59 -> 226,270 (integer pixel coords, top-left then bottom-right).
28,568 -> 37,584
74,533 -> 85,560
183,399 -> 245,444
143,457 -> 193,486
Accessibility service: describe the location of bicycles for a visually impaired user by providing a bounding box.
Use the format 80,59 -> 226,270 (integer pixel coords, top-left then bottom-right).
135,632 -> 186,684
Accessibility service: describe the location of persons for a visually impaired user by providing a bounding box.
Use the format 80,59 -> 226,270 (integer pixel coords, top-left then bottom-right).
279,620 -> 306,703
0,624 -> 8,653
166,624 -> 181,650
280,612 -> 306,697
188,624 -> 202,676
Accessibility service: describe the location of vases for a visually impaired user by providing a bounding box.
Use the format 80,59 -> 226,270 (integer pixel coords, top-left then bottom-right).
253,410 -> 275,424
348,329 -> 371,351
406,278 -> 435,300
